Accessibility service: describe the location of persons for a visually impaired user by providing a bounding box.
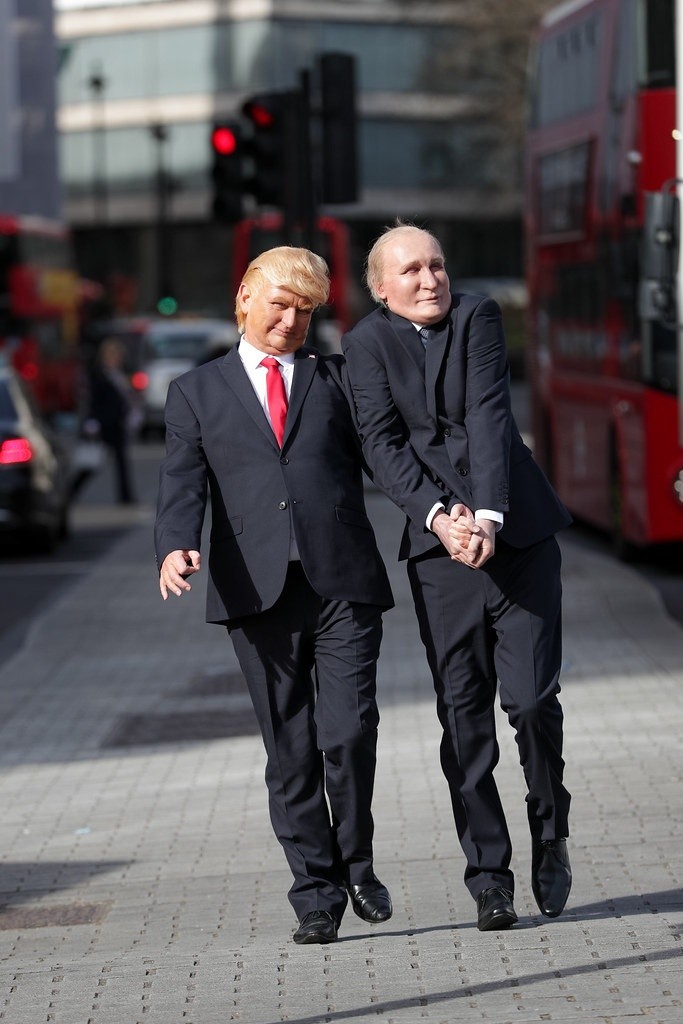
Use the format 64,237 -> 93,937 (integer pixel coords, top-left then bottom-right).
88,337 -> 141,505
342,224 -> 573,932
155,247 -> 476,944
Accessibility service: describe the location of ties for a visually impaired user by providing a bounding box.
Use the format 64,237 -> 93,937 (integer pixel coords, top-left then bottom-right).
419,325 -> 429,351
260,357 -> 289,450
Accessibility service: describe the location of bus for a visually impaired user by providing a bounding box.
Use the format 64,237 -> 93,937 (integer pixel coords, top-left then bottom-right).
0,214 -> 98,416
510,0 -> 683,545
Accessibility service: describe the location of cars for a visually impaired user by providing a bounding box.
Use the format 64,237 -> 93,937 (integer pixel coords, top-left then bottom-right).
135,317 -> 237,429
0,366 -> 75,558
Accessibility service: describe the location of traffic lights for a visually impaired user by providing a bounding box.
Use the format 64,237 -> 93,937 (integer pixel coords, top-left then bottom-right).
246,93 -> 302,225
305,50 -> 366,207
203,117 -> 243,227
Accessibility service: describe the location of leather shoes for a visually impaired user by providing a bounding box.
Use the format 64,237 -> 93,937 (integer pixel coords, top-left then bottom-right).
347,873 -> 392,924
532,837 -> 572,917
294,911 -> 339,944
477,887 -> 519,931
